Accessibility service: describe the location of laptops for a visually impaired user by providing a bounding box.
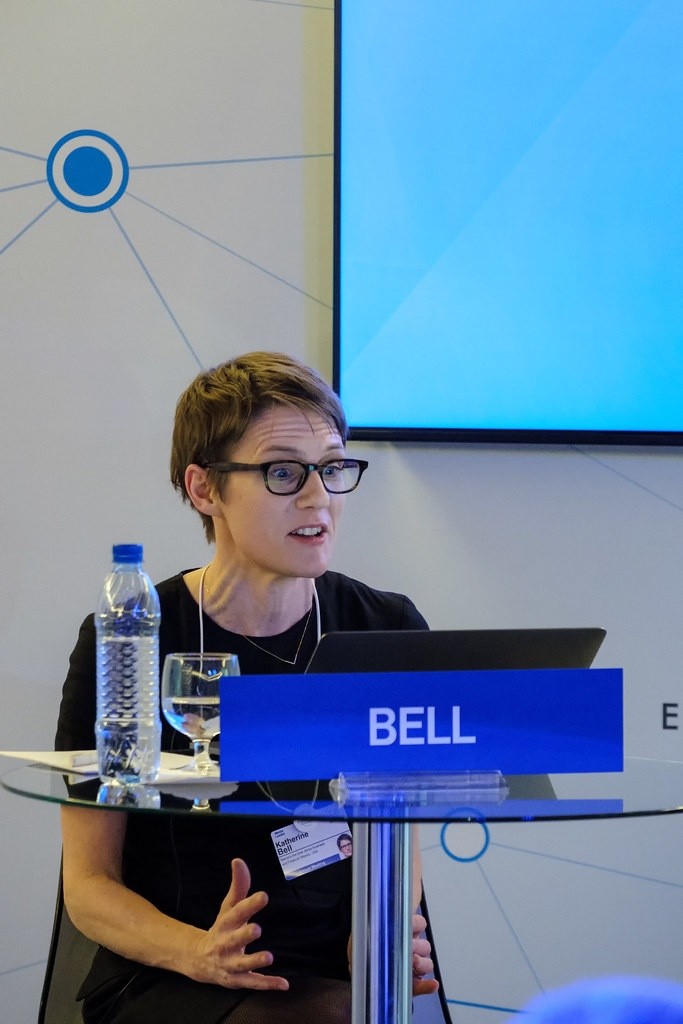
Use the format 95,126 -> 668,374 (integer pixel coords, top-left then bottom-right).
305,628 -> 608,674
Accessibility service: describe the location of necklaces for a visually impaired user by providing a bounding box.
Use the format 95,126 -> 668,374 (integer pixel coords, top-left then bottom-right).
195,568 -> 314,666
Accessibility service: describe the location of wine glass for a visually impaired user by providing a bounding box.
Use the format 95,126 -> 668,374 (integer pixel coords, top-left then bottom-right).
160,653 -> 240,775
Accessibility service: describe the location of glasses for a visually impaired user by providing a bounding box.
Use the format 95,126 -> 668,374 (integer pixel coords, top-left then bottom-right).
204,457 -> 370,496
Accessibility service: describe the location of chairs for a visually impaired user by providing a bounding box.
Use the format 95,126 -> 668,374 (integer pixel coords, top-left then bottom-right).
36,842 -> 452,1024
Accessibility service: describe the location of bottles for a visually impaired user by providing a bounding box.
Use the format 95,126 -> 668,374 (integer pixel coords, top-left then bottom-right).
94,544 -> 161,785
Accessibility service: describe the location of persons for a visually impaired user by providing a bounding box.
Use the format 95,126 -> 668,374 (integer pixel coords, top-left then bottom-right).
335,832 -> 355,858
35,351 -> 439,1024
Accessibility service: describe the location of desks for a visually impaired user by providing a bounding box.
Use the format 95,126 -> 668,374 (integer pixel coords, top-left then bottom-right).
0,757 -> 683,1024
